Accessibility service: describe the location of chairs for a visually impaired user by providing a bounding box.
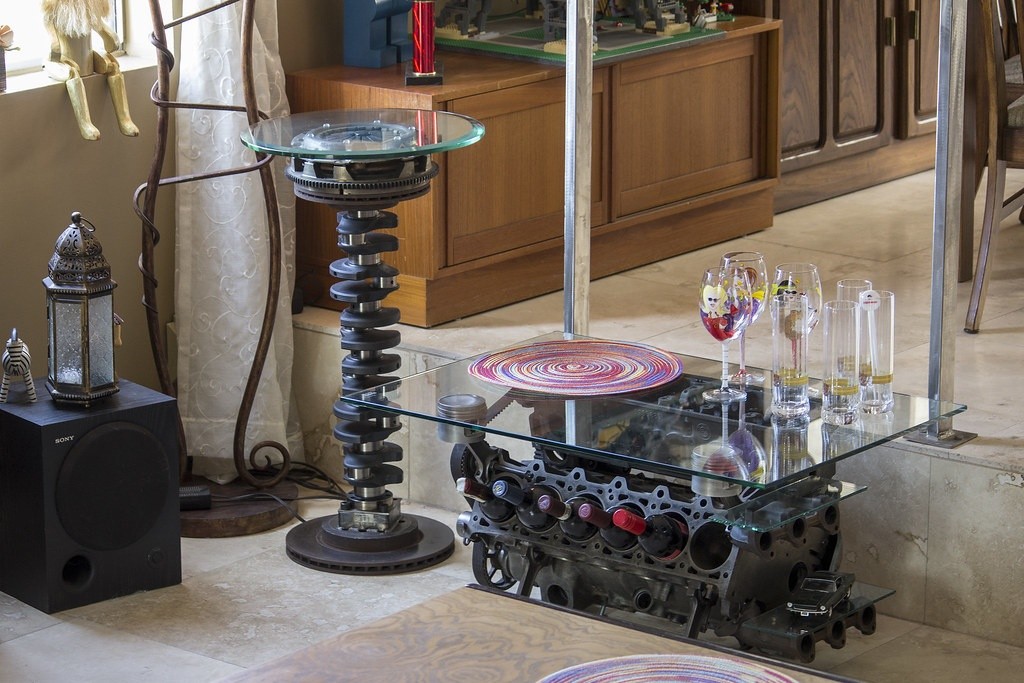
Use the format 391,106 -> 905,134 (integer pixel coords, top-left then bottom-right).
963,0 -> 1023,333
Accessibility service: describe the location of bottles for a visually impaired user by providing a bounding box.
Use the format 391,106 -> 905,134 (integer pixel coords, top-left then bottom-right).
537,494 -> 601,542
492,480 -> 562,533
612,509 -> 688,561
577,504 -> 643,550
456,477 -> 515,522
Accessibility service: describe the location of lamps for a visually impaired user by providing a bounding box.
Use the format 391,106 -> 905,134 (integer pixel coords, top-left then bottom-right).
43,212 -> 120,409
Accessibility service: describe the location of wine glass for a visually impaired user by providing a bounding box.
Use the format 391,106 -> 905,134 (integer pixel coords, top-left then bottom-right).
720,251 -> 769,384
770,262 -> 822,397
698,265 -> 752,402
729,385 -> 768,485
703,404 -> 749,494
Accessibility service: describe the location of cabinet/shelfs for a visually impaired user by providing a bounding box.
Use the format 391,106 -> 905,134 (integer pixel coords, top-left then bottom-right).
710,1 -> 940,212
285,14 -> 781,329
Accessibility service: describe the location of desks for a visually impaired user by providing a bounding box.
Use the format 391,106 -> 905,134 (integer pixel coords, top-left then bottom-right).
239,110 -> 486,574
338,329 -> 969,663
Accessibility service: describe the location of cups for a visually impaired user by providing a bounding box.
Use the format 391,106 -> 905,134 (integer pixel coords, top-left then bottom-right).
690,443 -> 733,497
857,290 -> 895,415
771,293 -> 811,416
820,299 -> 862,426
837,278 -> 871,384
